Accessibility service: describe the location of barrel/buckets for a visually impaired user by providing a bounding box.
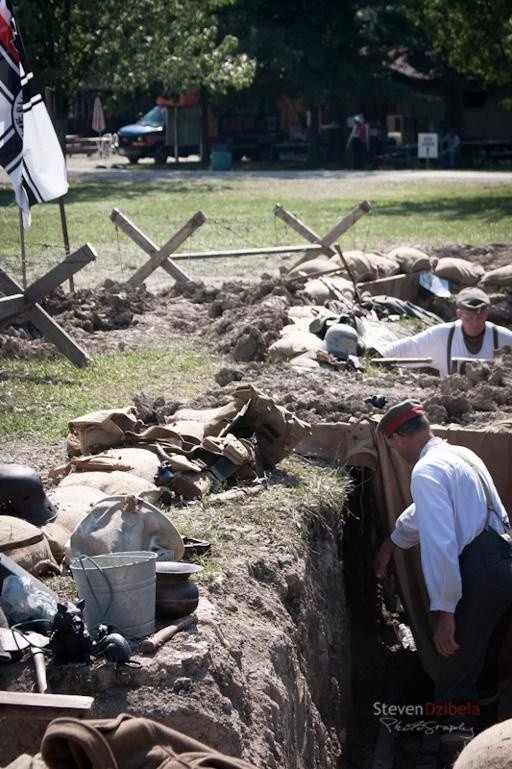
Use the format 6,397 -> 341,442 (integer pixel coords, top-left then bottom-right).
68,548 -> 160,642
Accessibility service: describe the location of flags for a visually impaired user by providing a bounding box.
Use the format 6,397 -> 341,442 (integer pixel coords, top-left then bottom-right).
0,1 -> 69,230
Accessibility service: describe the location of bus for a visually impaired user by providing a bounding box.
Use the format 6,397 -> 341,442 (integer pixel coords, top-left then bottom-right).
117,90 -> 306,167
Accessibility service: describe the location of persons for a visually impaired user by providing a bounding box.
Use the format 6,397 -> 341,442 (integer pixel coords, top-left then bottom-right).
436,128 -> 462,167
371,398 -> 511,769
345,113 -> 371,171
373,285 -> 511,380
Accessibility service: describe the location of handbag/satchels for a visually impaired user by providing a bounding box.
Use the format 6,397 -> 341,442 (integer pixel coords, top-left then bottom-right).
68,406 -> 139,456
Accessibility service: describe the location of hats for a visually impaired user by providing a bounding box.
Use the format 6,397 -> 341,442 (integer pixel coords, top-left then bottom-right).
376,399 -> 424,438
455,287 -> 489,314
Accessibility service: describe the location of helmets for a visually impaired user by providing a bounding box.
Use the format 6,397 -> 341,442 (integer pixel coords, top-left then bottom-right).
0,464 -> 57,524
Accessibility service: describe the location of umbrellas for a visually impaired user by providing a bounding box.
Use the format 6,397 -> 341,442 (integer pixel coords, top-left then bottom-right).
93,96 -> 107,159
